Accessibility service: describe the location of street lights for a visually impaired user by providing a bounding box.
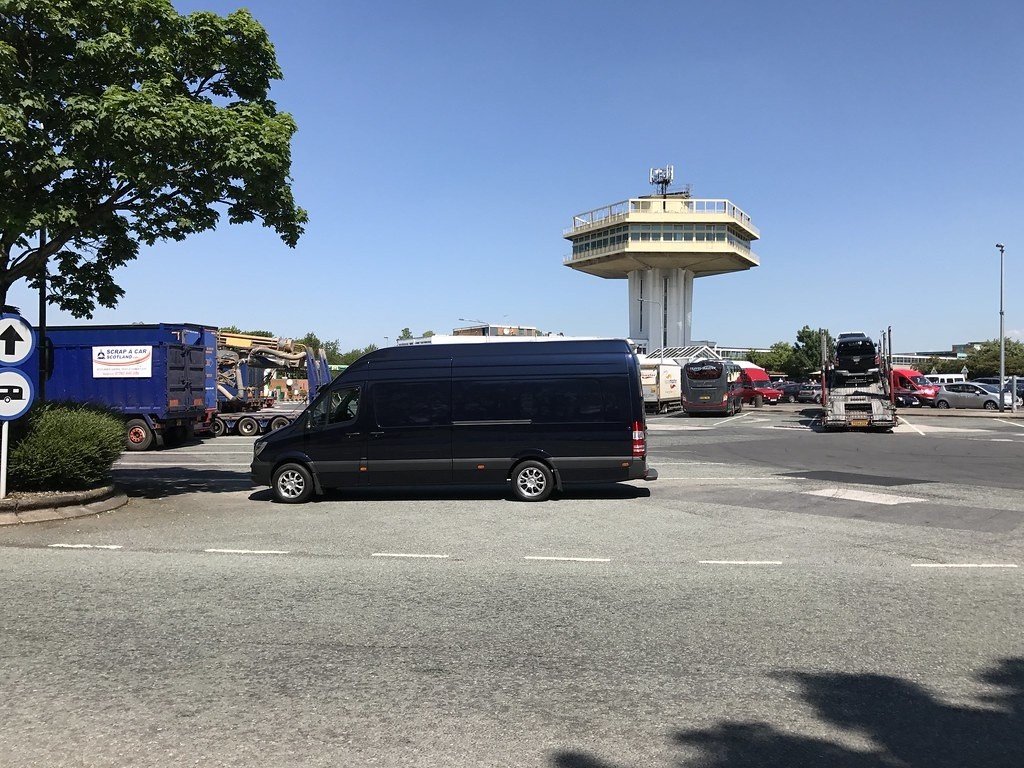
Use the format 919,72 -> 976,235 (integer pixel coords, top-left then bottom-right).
637,299 -> 663,365
458,315 -> 509,336
996,243 -> 1005,413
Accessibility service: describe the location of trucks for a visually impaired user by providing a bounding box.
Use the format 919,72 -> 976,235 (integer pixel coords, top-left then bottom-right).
820,326 -> 898,431
17,322 -> 218,451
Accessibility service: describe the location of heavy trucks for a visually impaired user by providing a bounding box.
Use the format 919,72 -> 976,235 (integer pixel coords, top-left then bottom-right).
195,327 -> 332,436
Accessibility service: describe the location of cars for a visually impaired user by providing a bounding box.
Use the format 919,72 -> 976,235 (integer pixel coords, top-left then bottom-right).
797,385 -> 822,404
972,377 -> 1001,388
933,382 -> 1021,410
1005,383 -> 1024,407
776,384 -> 804,403
832,332 -> 866,348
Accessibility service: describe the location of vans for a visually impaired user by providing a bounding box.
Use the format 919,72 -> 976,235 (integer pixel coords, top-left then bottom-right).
893,369 -> 940,407
924,374 -> 966,383
742,368 -> 783,406
249,338 -> 659,503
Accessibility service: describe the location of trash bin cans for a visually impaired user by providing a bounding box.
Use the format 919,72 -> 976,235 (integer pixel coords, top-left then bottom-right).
755,394 -> 763,408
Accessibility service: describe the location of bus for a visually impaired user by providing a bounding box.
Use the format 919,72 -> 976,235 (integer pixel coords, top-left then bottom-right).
680,360 -> 742,417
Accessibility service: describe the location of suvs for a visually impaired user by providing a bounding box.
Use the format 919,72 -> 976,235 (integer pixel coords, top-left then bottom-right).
834,339 -> 880,383
